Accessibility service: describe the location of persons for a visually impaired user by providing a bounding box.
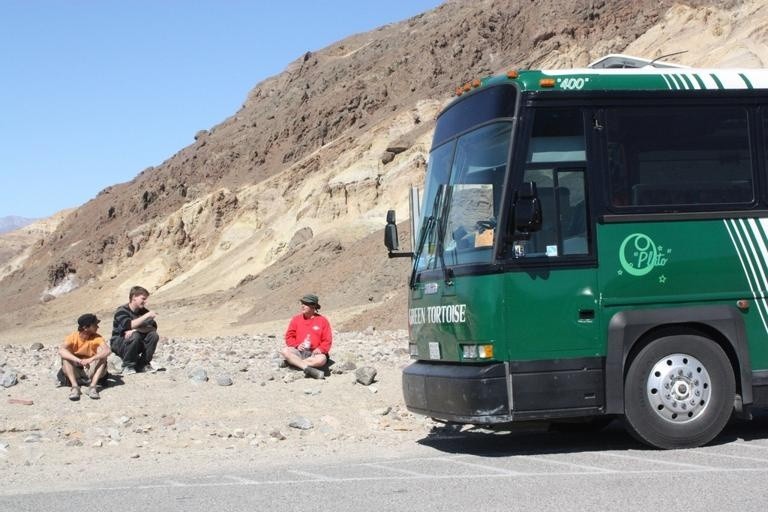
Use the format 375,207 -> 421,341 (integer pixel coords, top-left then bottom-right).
57,314 -> 112,399
279,294 -> 332,379
110,286 -> 159,376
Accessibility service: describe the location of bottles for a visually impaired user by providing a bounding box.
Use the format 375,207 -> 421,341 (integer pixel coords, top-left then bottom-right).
303,334 -> 312,352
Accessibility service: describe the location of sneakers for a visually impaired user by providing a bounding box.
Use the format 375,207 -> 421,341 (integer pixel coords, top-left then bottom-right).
279,360 -> 288,367
86,386 -> 99,398
138,364 -> 156,373
124,367 -> 136,374
305,367 -> 323,379
68,386 -> 80,399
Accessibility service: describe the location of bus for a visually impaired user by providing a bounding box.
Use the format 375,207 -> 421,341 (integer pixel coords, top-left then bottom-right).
381,54 -> 767,450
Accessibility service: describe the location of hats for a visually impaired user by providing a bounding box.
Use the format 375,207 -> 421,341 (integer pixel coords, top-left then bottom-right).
298,295 -> 317,304
78,314 -> 99,325
